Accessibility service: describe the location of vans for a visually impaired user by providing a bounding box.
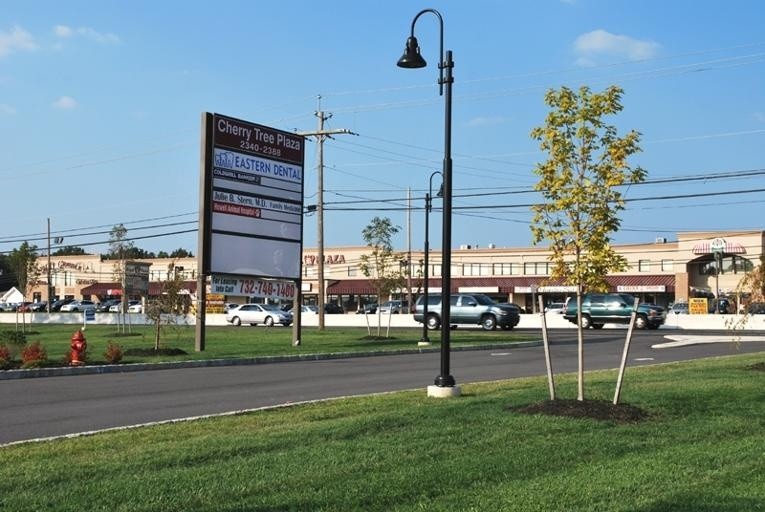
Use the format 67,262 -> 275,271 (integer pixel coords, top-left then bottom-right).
375,300 -> 409,314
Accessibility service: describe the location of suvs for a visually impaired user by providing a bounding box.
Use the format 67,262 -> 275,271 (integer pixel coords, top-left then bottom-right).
413,293 -> 520,331
563,292 -> 667,330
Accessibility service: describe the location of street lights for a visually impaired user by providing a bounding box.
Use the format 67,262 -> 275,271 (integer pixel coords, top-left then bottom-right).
418,171 -> 445,346
394,7 -> 463,400
714,251 -> 720,314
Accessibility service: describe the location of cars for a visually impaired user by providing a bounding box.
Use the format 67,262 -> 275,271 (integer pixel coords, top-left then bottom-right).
0,298 -> 163,314
355,304 -> 379,314
224,303 -> 343,327
672,298 -> 765,315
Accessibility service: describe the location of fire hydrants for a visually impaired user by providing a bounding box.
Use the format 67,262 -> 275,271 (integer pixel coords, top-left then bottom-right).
70,330 -> 87,366
